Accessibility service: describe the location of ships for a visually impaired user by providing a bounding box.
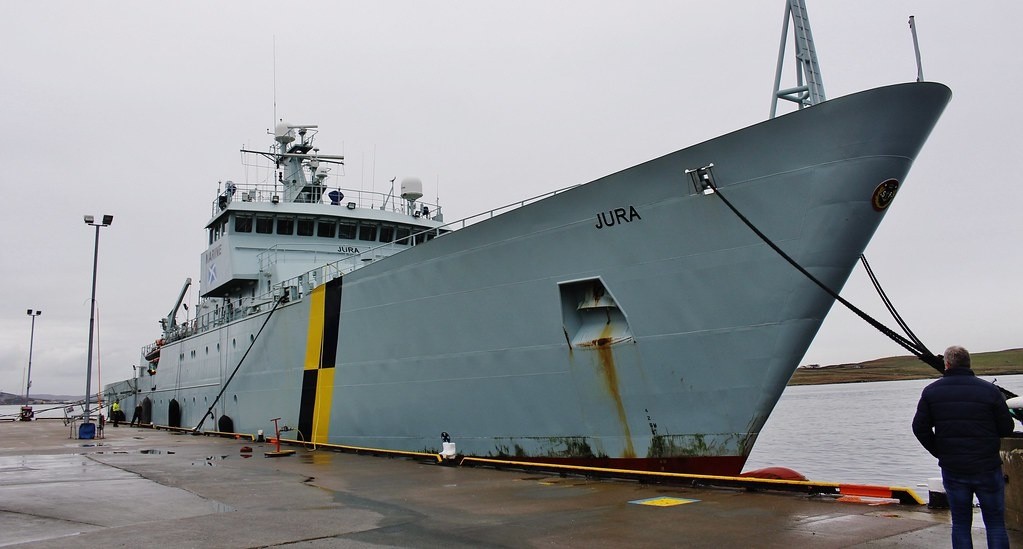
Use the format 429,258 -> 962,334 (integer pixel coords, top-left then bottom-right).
104,1 -> 954,476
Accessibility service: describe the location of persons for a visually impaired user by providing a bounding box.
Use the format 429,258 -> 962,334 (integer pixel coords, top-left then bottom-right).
130,406 -> 142,427
911,347 -> 1012,548
112,398 -> 120,428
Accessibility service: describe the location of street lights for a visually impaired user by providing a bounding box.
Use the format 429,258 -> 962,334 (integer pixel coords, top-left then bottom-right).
81,212 -> 115,437
24,308 -> 41,406
